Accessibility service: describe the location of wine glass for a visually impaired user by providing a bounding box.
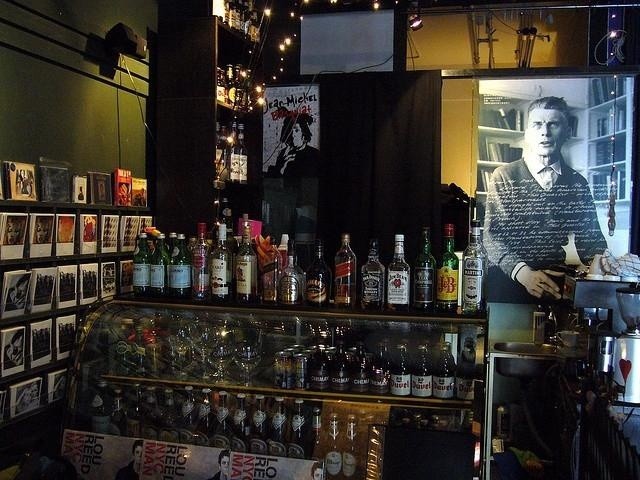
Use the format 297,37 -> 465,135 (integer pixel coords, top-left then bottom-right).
170,321 -> 261,388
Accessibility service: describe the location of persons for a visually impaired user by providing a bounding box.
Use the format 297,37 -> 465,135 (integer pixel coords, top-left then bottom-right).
205,450 -> 230,480
482,98 -> 609,306
114,440 -> 143,480
9,274 -> 29,308
16,169 -> 32,198
276,112 -> 320,179
311,462 -> 323,480
4,328 -> 24,366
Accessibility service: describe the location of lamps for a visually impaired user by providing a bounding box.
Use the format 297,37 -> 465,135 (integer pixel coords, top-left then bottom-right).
408,13 -> 423,28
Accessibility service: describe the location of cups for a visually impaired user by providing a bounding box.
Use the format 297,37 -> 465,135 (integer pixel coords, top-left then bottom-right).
556,330 -> 581,351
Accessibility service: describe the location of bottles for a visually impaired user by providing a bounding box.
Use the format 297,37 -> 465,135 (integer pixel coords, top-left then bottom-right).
401,410 -> 475,428
131,217 -> 491,319
495,399 -> 524,443
532,304 -> 556,346
89,378 -> 361,480
115,317 -> 161,381
216,0 -> 262,186
309,337 -> 482,403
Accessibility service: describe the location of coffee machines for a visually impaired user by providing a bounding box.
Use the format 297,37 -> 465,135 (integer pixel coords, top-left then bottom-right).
611,286 -> 640,408
541,263 -> 640,359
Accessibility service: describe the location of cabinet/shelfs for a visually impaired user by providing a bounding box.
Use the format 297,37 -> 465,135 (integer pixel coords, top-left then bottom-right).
153,0 -> 266,236
67,296 -> 494,479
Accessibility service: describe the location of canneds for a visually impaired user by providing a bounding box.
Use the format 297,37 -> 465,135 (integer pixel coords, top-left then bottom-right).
272,344 -> 374,393
